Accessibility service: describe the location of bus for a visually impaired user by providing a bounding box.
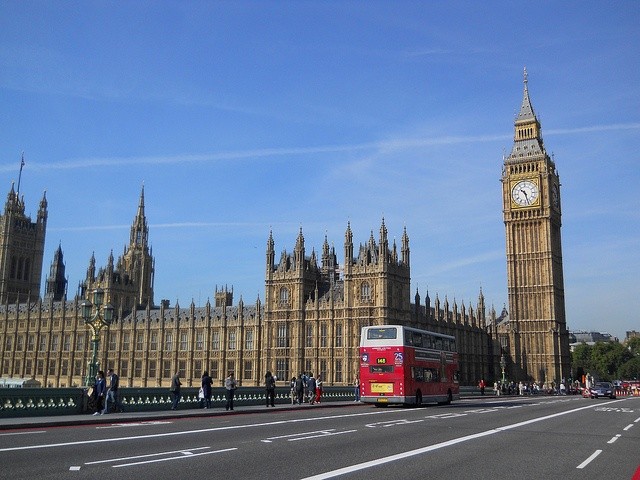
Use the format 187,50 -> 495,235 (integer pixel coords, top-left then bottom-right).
358,324 -> 460,406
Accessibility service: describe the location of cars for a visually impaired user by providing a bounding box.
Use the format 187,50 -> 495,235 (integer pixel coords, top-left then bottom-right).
590,382 -> 616,400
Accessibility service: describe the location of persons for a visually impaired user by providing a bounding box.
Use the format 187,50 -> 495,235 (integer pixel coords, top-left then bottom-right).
289,372 -> 323,404
264,371 -> 277,408
200,371 -> 213,409
353,373 -> 360,402
478,378 -> 485,394
86,369 -> 106,414
224,372 -> 236,410
102,369 -> 122,412
494,379 -> 585,398
169,369 -> 181,411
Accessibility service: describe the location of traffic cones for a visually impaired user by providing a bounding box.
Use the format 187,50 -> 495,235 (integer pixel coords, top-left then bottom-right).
615,390 -> 629,397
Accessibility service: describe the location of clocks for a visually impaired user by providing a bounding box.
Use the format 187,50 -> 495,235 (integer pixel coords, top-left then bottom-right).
511,180 -> 538,206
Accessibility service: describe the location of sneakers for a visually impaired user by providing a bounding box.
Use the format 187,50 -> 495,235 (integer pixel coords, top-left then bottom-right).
121,406 -> 124,413
101,409 -> 105,415
93,412 -> 101,416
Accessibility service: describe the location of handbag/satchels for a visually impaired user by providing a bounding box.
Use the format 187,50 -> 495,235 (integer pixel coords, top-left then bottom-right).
198,387 -> 204,398
231,384 -> 237,390
87,387 -> 95,398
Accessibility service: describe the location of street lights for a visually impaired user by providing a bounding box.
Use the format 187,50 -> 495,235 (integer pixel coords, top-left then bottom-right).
500,354 -> 507,384
79,282 -> 116,387
